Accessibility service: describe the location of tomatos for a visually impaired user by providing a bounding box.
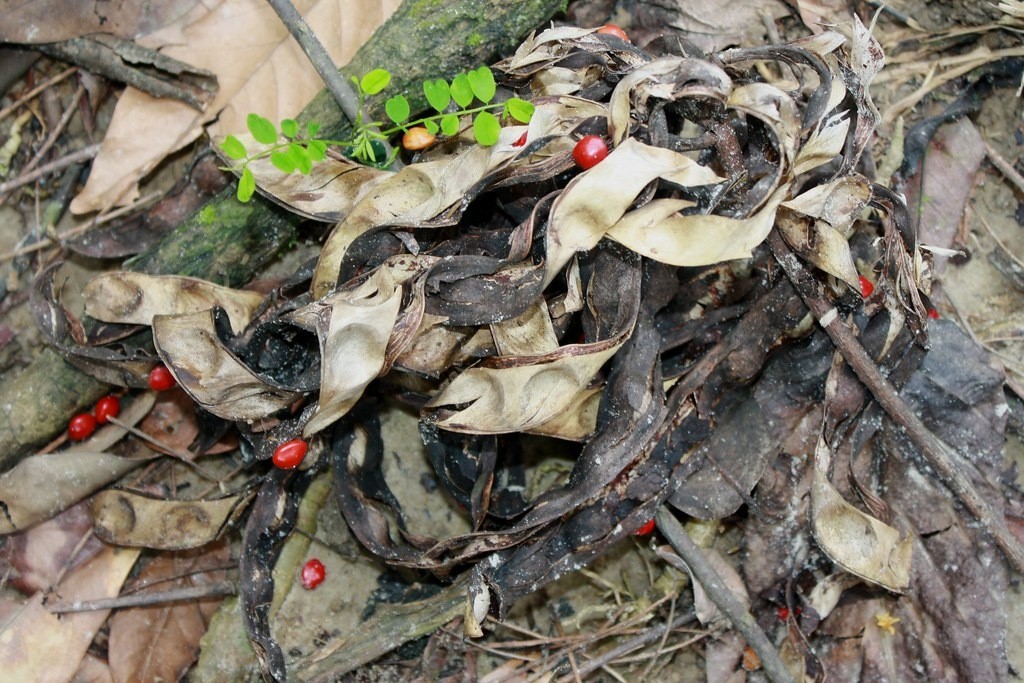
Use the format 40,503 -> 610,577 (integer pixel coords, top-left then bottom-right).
573,134 -> 608,168
858,274 -> 873,298
96,396 -> 120,423
302,558 -> 325,588
68,413 -> 97,441
149,367 -> 177,391
597,24 -> 629,45
272,439 -> 307,470
